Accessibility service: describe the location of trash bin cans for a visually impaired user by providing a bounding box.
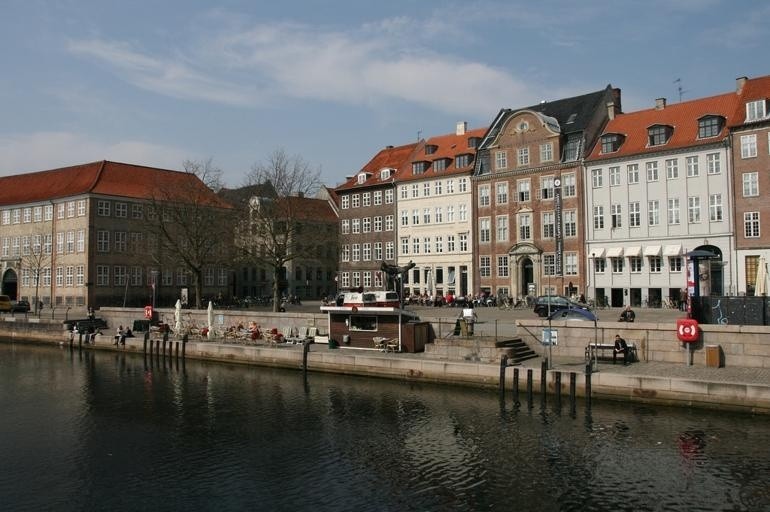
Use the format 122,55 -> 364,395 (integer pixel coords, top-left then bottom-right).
464,318 -> 474,335
706,345 -> 721,369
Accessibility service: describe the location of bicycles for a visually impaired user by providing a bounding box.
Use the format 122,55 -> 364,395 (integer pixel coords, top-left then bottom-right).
597,296 -> 611,310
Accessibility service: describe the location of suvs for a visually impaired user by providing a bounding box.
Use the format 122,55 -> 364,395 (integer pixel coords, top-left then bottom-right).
533,295 -> 593,318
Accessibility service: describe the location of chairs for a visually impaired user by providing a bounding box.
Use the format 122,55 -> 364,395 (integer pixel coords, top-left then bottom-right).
386,336 -> 401,354
373,335 -> 387,354
150,321 -> 317,346
405,294 -> 526,310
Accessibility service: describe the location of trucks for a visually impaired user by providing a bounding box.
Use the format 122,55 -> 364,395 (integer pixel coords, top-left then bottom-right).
320,288 -> 400,314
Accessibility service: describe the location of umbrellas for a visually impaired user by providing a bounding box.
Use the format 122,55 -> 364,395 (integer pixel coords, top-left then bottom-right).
208,301 -> 216,340
174,300 -> 183,340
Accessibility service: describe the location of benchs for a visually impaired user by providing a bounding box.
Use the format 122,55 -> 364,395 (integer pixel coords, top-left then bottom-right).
585,337 -> 636,365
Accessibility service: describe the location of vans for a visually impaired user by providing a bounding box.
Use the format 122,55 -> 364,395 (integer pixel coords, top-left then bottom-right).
0,295 -> 11,314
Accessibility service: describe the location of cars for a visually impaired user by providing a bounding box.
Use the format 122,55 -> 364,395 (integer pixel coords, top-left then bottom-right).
13,300 -> 30,312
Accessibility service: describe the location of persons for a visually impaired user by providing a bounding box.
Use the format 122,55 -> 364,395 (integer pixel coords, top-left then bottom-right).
404,289 -> 513,309
571,291 -> 587,305
612,335 -> 628,366
676,288 -> 687,312
68,322 -> 100,343
112,326 -> 135,346
318,296 -> 329,312
620,305 -> 635,322
39,300 -> 43,309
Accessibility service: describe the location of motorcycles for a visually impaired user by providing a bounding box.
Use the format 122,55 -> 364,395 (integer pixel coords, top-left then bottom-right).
404,292 -> 537,309
